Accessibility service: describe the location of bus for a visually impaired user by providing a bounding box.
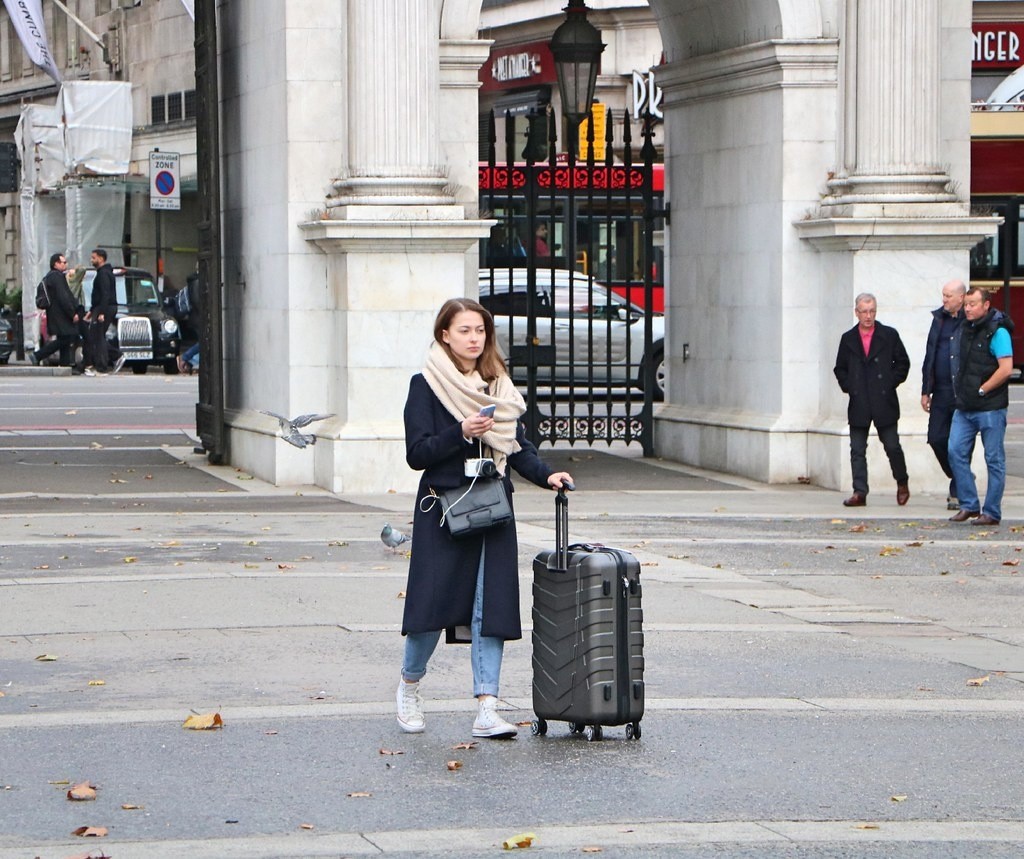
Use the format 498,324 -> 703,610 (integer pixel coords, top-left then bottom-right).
479,161 -> 668,306
968,104 -> 1024,369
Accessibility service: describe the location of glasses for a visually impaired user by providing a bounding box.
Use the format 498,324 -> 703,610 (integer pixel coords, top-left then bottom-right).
60,261 -> 66,264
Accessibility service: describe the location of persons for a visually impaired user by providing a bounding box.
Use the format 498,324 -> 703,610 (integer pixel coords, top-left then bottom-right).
947,286 -> 1016,526
521,222 -> 550,256
397,298 -> 574,737
921,281 -> 977,511
176,259 -> 200,373
833,292 -> 910,507
85,250 -> 125,375
29,253 -> 87,376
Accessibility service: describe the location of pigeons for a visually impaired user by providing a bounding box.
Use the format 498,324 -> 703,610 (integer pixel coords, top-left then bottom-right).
381,522 -> 412,554
252,409 -> 336,449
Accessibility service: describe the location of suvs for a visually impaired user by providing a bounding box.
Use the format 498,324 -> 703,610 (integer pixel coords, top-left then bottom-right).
480,267 -> 672,401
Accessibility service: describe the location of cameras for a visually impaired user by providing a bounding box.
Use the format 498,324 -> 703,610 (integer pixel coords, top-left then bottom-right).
464,457 -> 495,477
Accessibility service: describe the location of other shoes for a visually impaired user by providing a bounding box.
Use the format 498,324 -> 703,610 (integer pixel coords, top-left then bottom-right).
28,353 -> 38,366
947,496 -> 960,510
176,355 -> 187,373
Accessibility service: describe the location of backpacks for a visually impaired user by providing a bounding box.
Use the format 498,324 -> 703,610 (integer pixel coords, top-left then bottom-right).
172,284 -> 194,317
36,271 -> 55,309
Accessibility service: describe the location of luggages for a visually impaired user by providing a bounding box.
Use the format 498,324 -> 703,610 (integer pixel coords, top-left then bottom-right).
532,483 -> 645,740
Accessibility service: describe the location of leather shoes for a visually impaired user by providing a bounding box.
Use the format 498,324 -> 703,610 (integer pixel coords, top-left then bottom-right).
897,484 -> 909,504
948,509 -> 979,523
844,492 -> 867,506
971,514 -> 999,526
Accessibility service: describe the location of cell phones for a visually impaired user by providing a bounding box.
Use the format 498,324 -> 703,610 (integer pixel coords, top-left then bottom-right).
480,404 -> 496,419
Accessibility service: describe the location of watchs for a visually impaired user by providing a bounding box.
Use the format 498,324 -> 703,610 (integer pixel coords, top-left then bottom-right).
979,388 -> 985,396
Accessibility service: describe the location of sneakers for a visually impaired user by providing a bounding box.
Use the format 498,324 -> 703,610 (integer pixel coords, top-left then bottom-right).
113,355 -> 126,373
85,369 -> 107,377
394,676 -> 426,731
472,697 -> 518,739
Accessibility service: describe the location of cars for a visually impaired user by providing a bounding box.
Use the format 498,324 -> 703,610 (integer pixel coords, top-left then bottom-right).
0,317 -> 14,360
63,268 -> 182,374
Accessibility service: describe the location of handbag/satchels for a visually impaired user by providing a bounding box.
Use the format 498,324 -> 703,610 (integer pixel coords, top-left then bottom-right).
441,477 -> 513,534
